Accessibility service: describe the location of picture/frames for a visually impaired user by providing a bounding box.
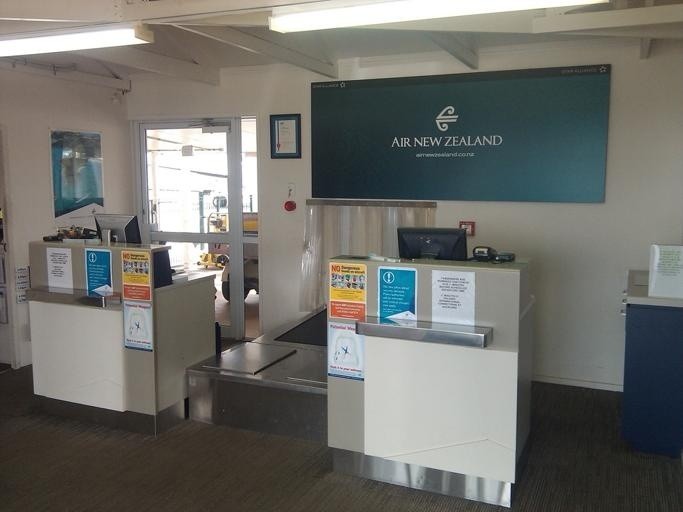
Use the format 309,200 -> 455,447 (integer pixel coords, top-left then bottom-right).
270,114 -> 301,159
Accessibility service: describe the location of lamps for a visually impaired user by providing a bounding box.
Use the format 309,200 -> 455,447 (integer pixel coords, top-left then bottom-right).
0,22 -> 156,58
269,0 -> 607,34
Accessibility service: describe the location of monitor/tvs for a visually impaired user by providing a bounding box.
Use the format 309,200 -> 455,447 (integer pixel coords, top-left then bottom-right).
94,214 -> 142,244
397,228 -> 467,261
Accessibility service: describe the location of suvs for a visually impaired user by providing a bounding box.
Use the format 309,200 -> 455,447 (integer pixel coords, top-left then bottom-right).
221,231 -> 259,302
